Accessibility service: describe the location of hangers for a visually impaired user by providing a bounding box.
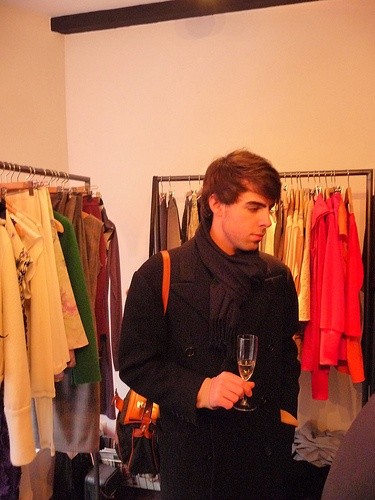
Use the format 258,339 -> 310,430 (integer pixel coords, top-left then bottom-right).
160,169 -> 351,201
0,161 -> 103,205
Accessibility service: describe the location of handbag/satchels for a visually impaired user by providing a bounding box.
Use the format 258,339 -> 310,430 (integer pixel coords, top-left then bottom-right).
112,389 -> 160,475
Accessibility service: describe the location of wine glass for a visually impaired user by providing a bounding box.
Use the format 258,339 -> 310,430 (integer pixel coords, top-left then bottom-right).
234,334 -> 257,411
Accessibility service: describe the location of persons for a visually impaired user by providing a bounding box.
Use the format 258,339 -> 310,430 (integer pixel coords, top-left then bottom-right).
118,149 -> 302,500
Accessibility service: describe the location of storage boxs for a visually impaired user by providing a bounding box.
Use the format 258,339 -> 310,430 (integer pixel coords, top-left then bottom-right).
100,448 -> 161,492
